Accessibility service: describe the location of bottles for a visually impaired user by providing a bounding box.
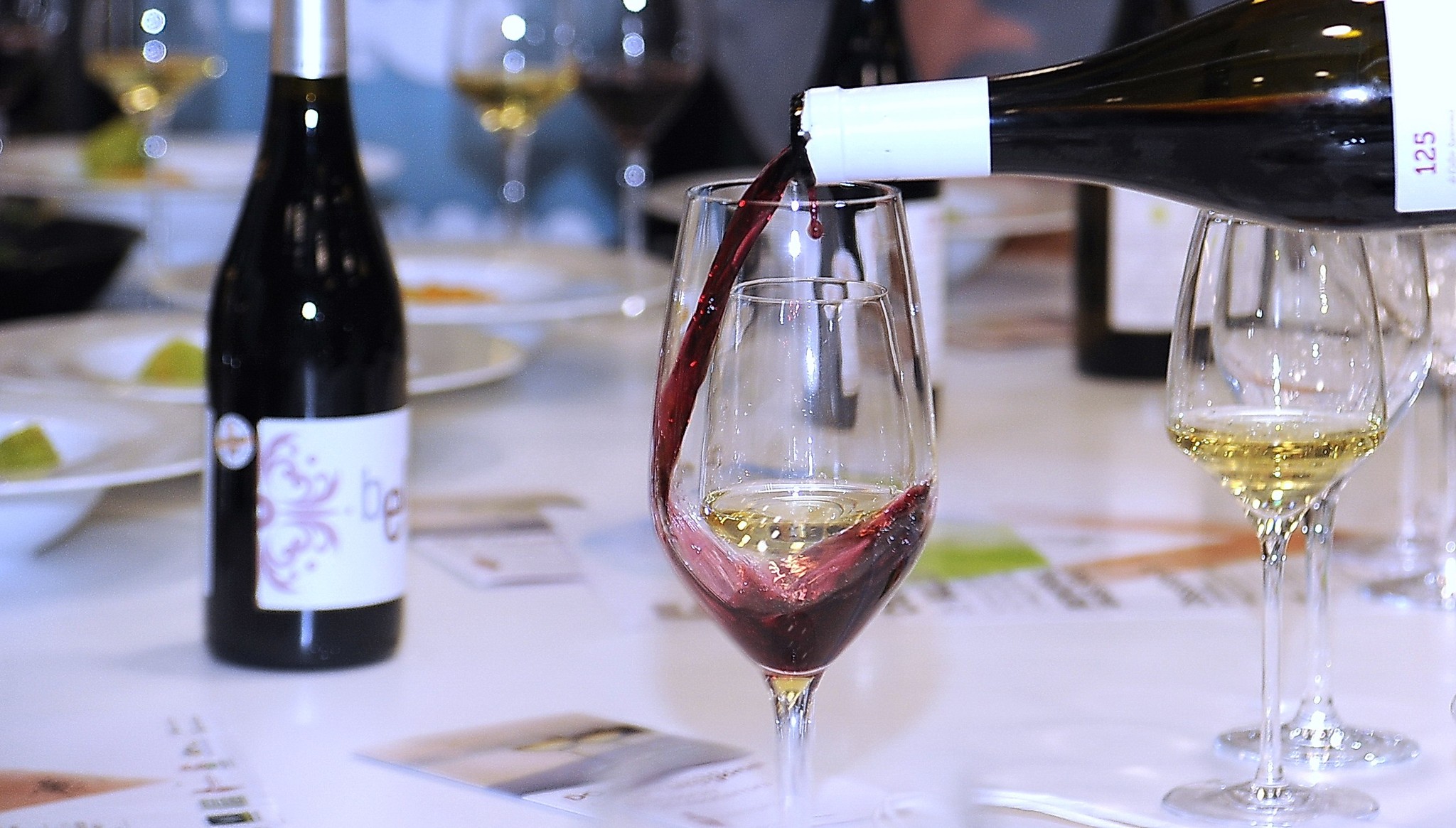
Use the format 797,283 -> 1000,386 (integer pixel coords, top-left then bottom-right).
204,0 -> 416,672
1069,2 -> 1237,379
780,2 -> 954,430
792,0 -> 1455,237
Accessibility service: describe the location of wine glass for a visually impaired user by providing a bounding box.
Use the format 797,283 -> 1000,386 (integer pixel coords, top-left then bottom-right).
69,0 -> 224,155
1160,192 -> 1456,822
448,1 -> 587,238
574,0 -> 708,262
650,185 -> 961,827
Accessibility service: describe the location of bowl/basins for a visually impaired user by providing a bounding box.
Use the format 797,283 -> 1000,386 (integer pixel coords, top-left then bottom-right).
0,197 -> 144,315
639,170 -> 1077,288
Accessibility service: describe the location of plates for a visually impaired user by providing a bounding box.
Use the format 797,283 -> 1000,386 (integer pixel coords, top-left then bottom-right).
0,305 -> 530,414
134,245 -> 670,319
0,132 -> 400,233
0,372 -> 214,560
